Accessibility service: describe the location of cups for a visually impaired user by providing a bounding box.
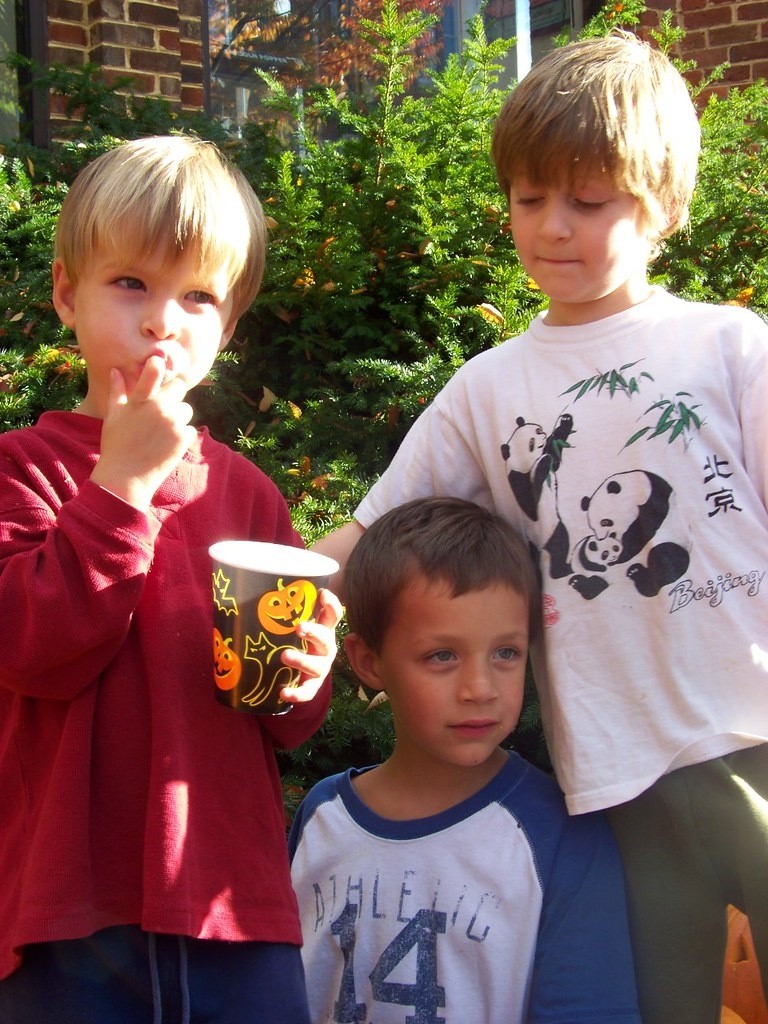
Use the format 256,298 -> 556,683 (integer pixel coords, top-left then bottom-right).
209,540 -> 340,716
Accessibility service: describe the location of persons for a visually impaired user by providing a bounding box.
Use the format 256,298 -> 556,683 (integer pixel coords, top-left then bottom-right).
308,27 -> 768,1024
282,494 -> 644,1024
0,133 -> 348,1024
